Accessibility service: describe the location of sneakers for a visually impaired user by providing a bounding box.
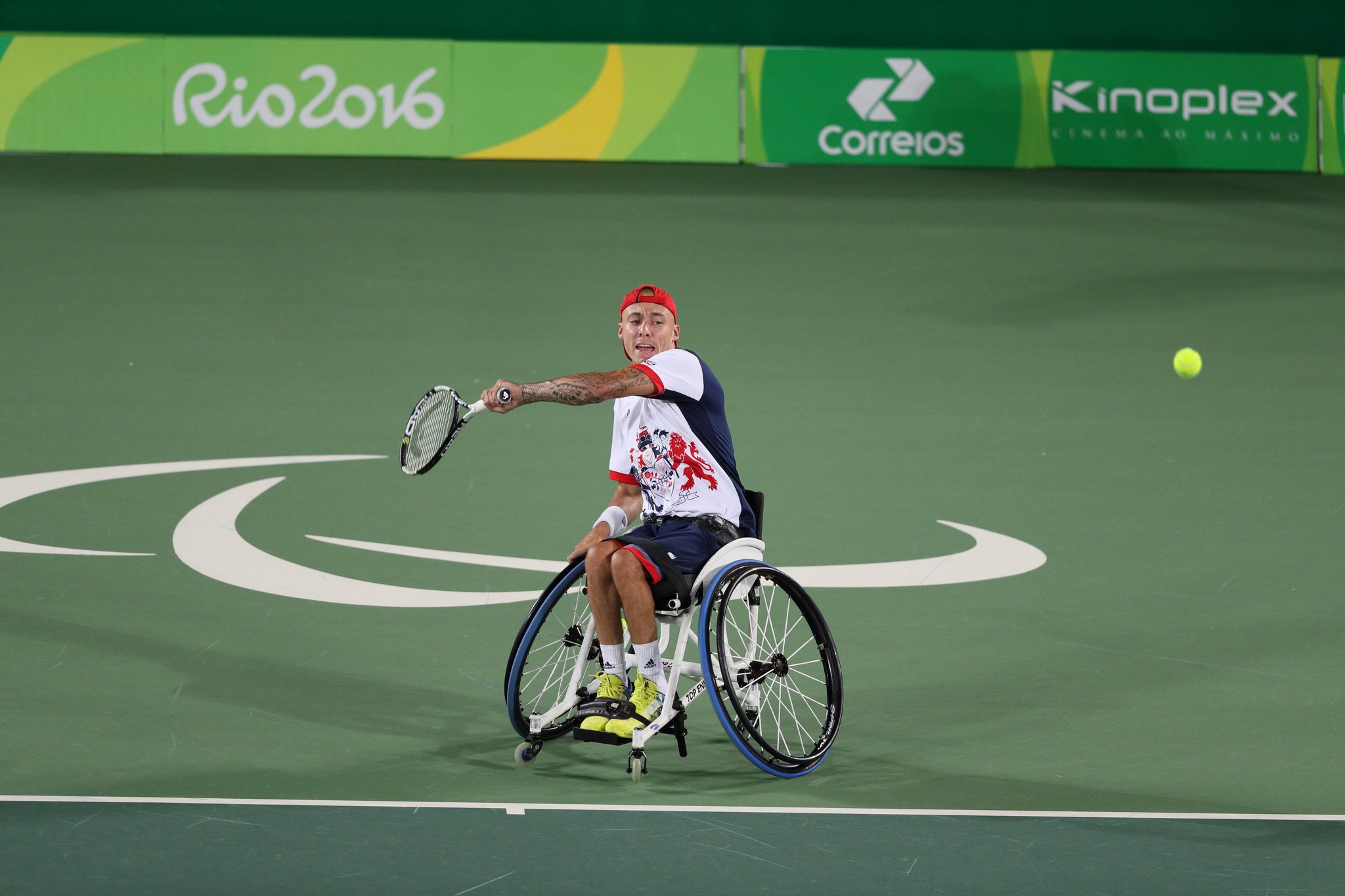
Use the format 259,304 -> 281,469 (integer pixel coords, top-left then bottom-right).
605,668 -> 664,739
579,670 -> 633,733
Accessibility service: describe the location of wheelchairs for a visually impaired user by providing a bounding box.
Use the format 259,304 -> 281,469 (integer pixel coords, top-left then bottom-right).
503,489 -> 845,786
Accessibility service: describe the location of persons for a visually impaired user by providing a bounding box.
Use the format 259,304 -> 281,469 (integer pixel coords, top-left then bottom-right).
480,286 -> 755,737
592,506 -> 628,537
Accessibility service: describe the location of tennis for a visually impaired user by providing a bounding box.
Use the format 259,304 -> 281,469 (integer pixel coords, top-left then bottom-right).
1173,347 -> 1202,379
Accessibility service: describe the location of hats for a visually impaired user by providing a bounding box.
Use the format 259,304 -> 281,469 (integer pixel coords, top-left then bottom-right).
618,281 -> 676,360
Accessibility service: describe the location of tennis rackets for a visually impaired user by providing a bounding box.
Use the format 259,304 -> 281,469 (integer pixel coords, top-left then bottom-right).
399,385 -> 512,476
